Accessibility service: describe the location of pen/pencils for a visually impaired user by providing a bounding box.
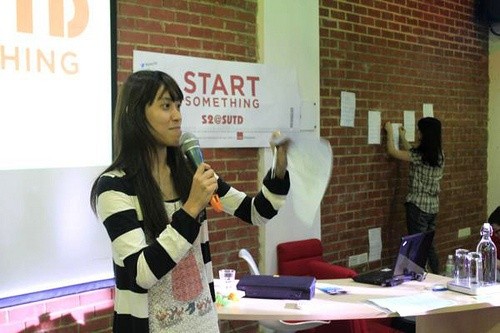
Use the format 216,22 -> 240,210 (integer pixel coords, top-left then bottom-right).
270,146 -> 278,179
433,288 -> 449,291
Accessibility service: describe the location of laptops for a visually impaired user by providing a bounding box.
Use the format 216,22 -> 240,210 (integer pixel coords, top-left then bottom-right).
351,229 -> 435,288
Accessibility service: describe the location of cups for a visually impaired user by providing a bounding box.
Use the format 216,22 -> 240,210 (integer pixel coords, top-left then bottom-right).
465,251 -> 483,288
454,250 -> 470,285
218,269 -> 235,283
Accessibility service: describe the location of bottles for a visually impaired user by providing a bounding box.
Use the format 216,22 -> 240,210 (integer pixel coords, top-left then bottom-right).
475,223 -> 497,286
445,254 -> 455,278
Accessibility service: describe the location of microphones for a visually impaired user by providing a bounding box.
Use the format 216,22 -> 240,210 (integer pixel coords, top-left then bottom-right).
181,132 -> 223,212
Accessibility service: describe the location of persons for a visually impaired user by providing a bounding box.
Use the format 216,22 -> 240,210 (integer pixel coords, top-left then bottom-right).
488,205 -> 500,270
90,70 -> 290,333
385,116 -> 445,274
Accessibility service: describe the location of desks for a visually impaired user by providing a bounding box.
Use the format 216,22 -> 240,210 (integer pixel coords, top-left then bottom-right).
214,273 -> 500,333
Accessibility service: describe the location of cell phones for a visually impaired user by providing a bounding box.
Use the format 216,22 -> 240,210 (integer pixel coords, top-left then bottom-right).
448,280 -> 479,294
